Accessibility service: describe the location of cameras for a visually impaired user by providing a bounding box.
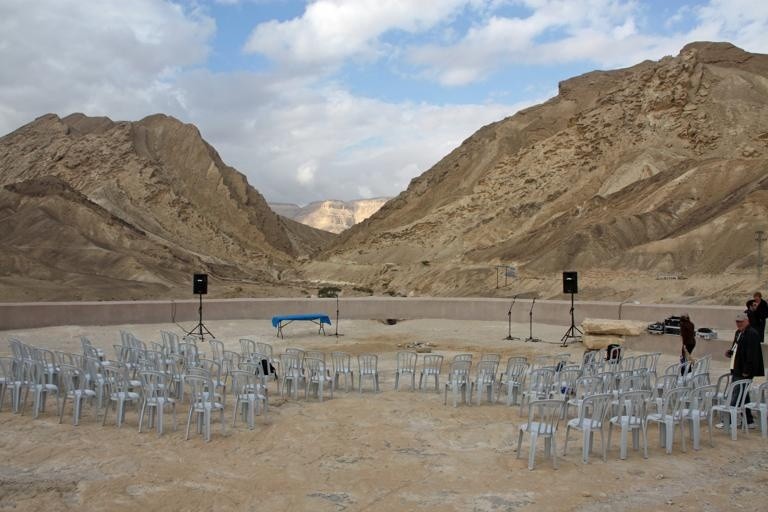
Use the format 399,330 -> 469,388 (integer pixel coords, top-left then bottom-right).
728,350 -> 733,355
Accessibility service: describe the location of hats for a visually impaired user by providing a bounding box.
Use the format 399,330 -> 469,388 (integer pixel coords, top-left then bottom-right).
735,313 -> 750,322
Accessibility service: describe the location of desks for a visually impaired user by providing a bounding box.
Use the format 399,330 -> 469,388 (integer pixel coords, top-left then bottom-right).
267,312 -> 332,340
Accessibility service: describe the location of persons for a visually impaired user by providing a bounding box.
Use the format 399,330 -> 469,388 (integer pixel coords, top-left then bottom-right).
745,300 -> 761,331
718,313 -> 764,432
678,311 -> 695,378
753,292 -> 767,342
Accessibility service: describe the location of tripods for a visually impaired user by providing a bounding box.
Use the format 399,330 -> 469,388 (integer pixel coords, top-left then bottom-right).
328,309 -> 344,339
525,311 -> 541,342
501,310 -> 519,340
561,293 -> 583,346
182,293 -> 216,342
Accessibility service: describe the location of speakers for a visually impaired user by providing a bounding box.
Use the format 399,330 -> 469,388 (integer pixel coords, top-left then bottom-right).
193,273 -> 208,294
563,271 -> 578,294
607,344 -> 621,364
